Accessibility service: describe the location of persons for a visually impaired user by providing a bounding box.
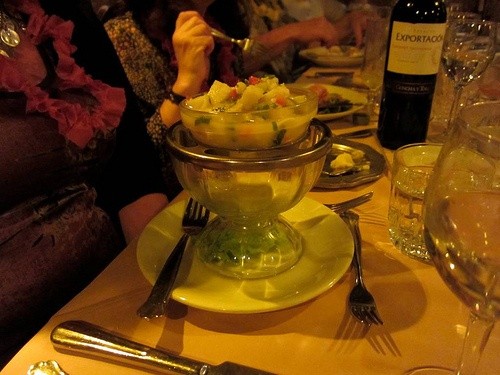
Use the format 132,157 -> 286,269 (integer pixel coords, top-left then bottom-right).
204,0 -> 382,88
87,0 -> 214,244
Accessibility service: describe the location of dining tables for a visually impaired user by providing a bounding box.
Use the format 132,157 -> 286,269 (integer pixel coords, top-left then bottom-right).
0,66 -> 500,375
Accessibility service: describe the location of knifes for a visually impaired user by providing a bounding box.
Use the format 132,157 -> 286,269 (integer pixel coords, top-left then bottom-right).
50,320 -> 280,375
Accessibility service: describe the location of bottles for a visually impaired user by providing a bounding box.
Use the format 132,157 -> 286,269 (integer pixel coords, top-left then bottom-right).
376,0 -> 447,150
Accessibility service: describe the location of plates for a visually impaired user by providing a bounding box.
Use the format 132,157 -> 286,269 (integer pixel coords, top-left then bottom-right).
315,136 -> 386,189
298,45 -> 365,67
301,83 -> 368,120
138,196 -> 355,314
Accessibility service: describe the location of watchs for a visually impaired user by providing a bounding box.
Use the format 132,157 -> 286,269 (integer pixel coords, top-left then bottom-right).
164,86 -> 187,105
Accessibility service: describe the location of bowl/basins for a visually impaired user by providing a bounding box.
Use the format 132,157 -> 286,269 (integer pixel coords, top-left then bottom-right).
179,84 -> 317,150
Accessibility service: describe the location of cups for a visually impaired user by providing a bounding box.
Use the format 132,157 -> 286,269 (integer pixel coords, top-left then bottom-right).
387,142 -> 443,264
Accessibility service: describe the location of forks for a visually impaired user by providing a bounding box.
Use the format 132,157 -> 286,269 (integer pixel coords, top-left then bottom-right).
137,197 -> 210,320
209,27 -> 270,64
340,211 -> 385,326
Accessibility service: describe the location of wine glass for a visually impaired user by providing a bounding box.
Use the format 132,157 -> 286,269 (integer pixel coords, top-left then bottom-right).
402,100 -> 500,375
427,19 -> 497,142
